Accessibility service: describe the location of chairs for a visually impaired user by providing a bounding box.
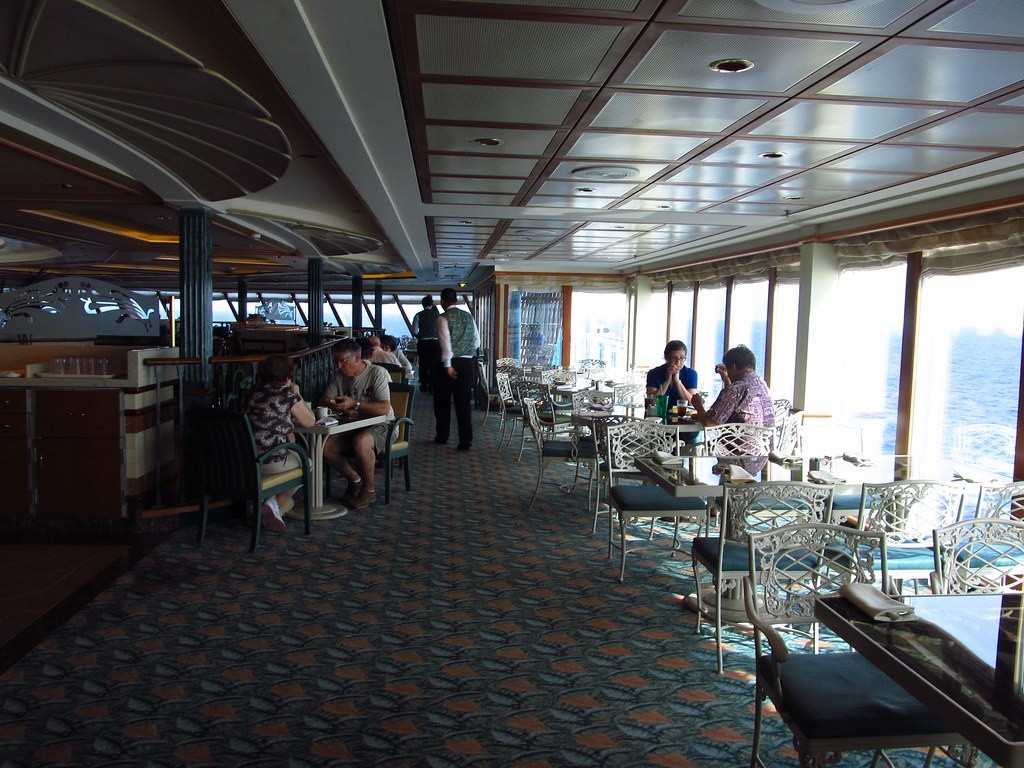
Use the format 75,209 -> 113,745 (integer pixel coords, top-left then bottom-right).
192,361 -> 417,553
478,358 -> 1024,768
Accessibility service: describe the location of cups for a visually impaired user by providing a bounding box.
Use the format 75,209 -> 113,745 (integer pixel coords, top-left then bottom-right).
318,406 -> 332,419
677,400 -> 689,418
644,394 -> 654,412
655,395 -> 669,417
48,354 -> 111,375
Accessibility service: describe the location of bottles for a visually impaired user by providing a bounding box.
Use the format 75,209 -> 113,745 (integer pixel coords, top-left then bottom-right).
408,339 -> 417,350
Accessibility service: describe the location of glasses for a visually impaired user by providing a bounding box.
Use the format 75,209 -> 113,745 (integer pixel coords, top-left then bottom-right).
332,354 -> 354,367
667,356 -> 686,362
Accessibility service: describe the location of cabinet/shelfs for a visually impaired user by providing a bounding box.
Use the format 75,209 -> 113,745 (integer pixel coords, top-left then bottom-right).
0,383 -> 128,542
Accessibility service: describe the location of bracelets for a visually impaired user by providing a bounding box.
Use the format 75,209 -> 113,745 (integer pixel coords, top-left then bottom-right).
674,379 -> 682,385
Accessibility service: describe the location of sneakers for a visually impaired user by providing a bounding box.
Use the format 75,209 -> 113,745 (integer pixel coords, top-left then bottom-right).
349,489 -> 376,509
340,477 -> 363,504
263,497 -> 286,531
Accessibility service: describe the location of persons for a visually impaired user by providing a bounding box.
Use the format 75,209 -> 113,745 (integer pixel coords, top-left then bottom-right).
320,338 -> 400,509
413,295 -> 442,392
244,353 -> 316,532
691,344 -> 775,518
367,335 -> 413,372
431,288 -> 481,451
646,340 -> 700,443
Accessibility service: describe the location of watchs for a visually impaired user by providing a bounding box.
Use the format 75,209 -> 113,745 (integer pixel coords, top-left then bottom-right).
355,401 -> 361,411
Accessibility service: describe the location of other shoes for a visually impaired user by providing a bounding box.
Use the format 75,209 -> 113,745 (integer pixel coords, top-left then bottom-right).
434,437 -> 447,444
420,385 -> 427,391
705,507 -> 717,518
458,443 -> 470,449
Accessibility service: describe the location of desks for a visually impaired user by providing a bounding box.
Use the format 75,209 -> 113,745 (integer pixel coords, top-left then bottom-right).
569,407 -> 708,513
523,379 -> 596,394
634,456 -> 885,626
286,410 -> 389,519
814,594 -> 1024,768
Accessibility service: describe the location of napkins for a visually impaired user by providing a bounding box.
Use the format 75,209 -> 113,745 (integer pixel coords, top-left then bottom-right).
807,470 -> 846,484
314,417 -> 337,426
577,410 -> 610,416
653,451 -> 683,464
769,450 -> 804,465
836,582 -> 918,623
843,452 -> 878,469
728,463 -> 756,485
645,417 -> 663,424
952,467 -> 978,483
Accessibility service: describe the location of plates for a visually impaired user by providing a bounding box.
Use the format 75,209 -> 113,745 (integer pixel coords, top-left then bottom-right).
0,370 -> 25,377
668,408 -> 691,413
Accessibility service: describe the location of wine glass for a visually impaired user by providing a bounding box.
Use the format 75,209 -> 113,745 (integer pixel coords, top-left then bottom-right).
399,335 -> 409,349
